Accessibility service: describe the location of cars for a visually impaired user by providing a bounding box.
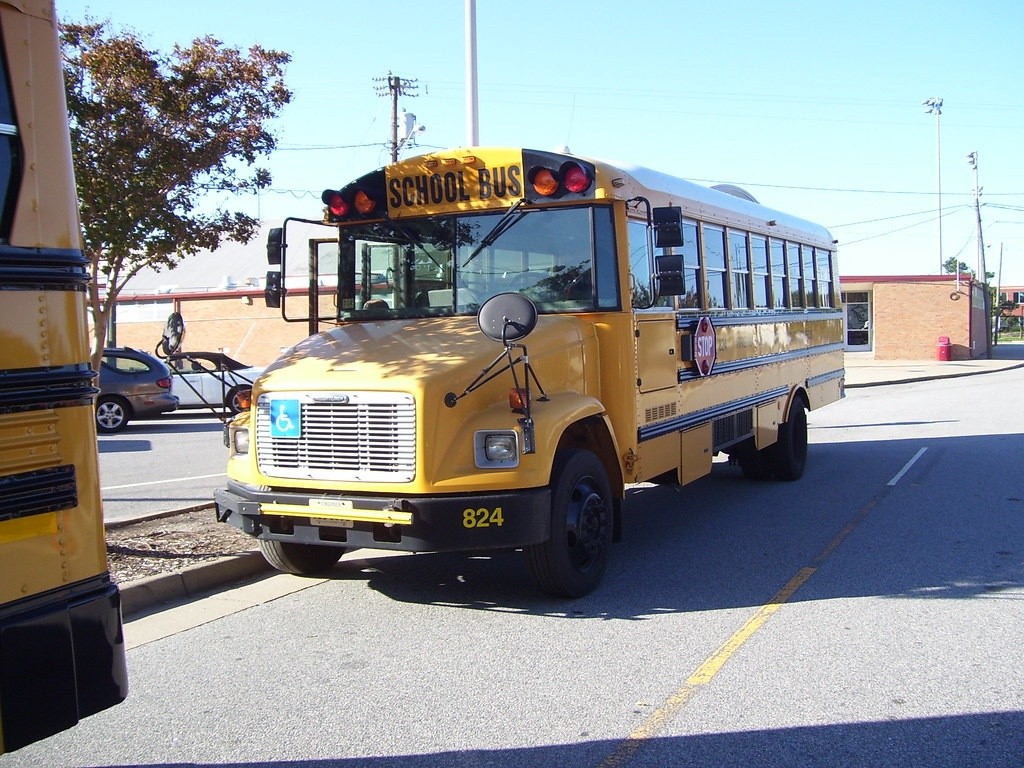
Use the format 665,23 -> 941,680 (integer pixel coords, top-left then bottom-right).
86,345 -> 179,432
143,352 -> 269,416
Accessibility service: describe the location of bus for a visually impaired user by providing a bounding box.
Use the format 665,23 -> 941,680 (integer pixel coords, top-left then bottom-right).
215,142 -> 852,609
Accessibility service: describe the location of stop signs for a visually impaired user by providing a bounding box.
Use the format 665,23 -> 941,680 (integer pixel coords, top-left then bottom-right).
693,316 -> 717,377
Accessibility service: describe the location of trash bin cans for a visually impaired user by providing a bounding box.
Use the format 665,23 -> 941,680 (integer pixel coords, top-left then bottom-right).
937,336 -> 953,361
